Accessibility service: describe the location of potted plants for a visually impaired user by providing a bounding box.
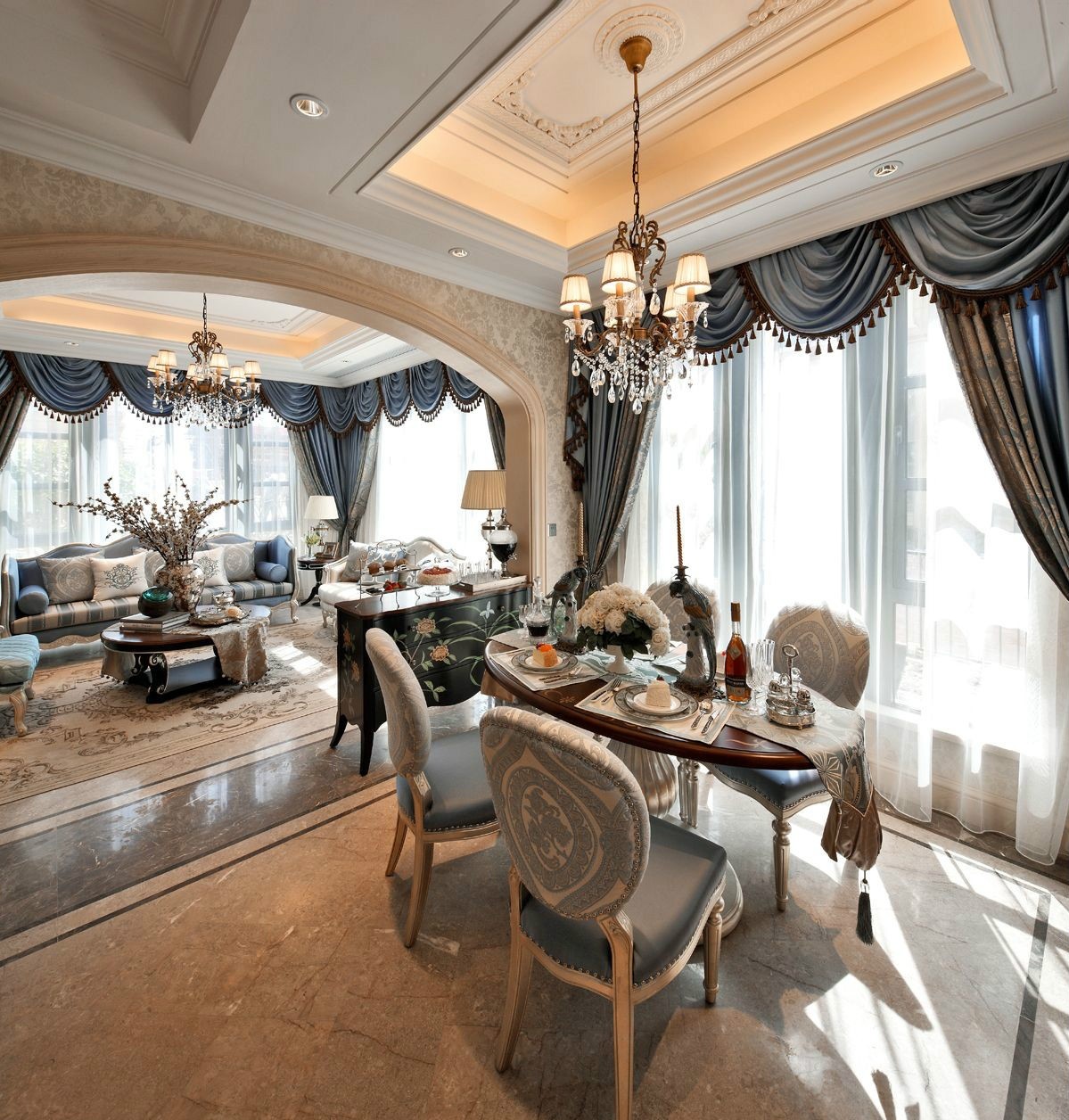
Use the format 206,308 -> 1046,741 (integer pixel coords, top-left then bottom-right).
304,533 -> 321,560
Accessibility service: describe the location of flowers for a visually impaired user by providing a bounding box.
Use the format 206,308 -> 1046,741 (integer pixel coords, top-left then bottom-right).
47,469 -> 252,560
574,584 -> 671,657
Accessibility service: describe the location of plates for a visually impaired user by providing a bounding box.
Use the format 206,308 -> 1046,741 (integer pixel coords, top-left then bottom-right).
624,687 -> 689,716
511,649 -> 578,674
614,684 -> 699,721
518,649 -> 569,670
716,653 -> 725,675
190,606 -> 252,625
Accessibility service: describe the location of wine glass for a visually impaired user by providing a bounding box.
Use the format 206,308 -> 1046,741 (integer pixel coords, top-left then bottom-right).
744,639 -> 774,716
519,603 -> 536,639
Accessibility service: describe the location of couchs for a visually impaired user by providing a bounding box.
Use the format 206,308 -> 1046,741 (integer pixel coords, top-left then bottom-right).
318,537 -> 467,643
0,532 -> 299,650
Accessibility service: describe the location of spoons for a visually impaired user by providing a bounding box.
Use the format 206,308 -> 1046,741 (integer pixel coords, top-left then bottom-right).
538,667 -> 583,681
601,687 -> 620,704
691,698 -> 712,728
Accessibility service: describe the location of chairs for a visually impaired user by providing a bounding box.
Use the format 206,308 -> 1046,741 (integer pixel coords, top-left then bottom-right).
687,606 -> 871,911
0,633 -> 41,736
477,706 -> 729,1120
641,577 -> 721,651
364,626 -> 505,949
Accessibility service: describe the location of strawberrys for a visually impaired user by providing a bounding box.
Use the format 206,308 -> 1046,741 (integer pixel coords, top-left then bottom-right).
383,580 -> 407,591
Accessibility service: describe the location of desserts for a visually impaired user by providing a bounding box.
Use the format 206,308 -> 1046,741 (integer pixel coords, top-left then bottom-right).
647,674 -> 671,708
533,642 -> 558,667
367,558 -> 407,574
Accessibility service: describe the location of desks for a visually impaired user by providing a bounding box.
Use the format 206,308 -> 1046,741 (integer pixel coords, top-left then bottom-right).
100,604 -> 272,704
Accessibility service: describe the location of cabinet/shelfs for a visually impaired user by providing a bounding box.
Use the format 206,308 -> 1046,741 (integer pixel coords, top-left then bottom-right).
359,539 -> 421,608
331,574 -> 534,776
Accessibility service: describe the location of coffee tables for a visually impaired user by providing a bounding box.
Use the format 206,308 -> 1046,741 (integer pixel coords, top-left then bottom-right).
296,554 -> 343,607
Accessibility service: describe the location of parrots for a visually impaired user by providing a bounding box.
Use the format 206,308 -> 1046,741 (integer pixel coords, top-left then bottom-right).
545,568 -> 587,623
670,581 -> 717,683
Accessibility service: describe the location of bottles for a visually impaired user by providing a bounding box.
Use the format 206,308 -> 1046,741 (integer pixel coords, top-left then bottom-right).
724,602 -> 752,704
490,507 -> 518,579
526,577 -> 551,644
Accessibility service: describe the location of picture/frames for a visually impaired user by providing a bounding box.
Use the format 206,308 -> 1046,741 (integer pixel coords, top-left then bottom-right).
322,542 -> 339,559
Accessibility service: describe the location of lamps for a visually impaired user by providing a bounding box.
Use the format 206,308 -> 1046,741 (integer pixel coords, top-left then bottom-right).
461,469 -> 506,568
304,494 -> 339,559
147,292 -> 261,434
562,34 -> 713,414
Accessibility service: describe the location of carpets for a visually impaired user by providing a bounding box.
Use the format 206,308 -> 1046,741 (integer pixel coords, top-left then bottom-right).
0,612 -> 360,845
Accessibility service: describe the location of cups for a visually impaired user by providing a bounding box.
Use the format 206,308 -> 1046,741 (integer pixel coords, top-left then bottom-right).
543,604 -> 566,637
458,562 -> 501,582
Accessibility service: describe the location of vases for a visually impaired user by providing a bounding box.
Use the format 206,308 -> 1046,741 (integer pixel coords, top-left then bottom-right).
602,644 -> 636,675
155,559 -> 206,611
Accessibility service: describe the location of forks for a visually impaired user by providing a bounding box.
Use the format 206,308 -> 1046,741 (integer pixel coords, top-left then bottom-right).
591,679 -> 622,701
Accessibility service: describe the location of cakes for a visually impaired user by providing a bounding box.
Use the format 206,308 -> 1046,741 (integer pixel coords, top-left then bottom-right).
417,566 -> 457,585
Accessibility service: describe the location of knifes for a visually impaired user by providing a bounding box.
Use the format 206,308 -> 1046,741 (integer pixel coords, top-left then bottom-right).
702,706 -> 724,733
544,674 -> 594,683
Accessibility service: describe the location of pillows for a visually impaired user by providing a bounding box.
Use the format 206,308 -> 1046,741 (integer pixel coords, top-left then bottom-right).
18,585 -> 49,615
340,539 -> 397,583
36,549 -> 106,606
205,540 -> 256,581
181,547 -> 230,586
356,545 -> 407,585
90,552 -> 149,602
255,561 -> 287,583
131,545 -> 167,587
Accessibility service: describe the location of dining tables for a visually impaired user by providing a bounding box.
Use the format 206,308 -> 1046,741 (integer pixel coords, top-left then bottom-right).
486,624 -> 885,947
